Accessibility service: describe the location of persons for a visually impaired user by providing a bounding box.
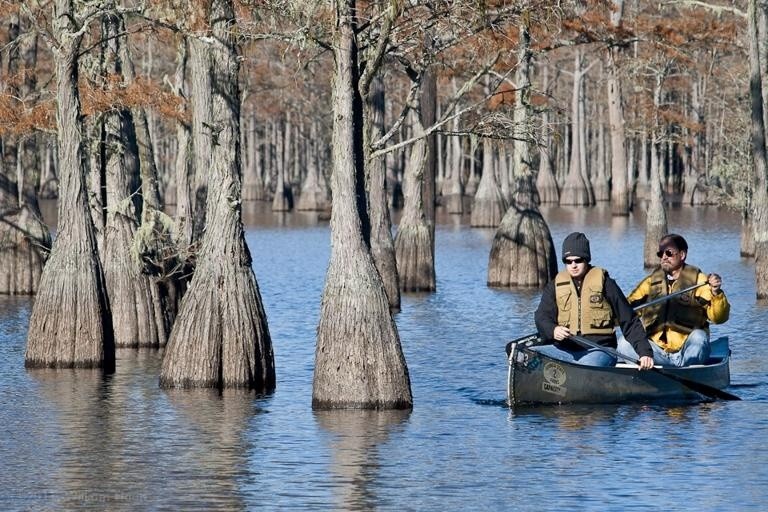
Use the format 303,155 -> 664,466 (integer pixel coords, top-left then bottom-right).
624,233 -> 731,365
531,231 -> 655,373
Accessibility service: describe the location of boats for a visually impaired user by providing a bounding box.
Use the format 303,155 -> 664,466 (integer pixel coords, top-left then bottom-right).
504,335 -> 732,410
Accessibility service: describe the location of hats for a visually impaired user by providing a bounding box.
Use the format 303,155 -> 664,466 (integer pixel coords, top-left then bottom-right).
562,232 -> 591,263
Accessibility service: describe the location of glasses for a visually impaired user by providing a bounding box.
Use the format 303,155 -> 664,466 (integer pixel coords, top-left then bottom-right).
563,258 -> 584,264
657,251 -> 672,257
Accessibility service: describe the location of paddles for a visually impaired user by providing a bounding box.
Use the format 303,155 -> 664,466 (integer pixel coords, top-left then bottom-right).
569,334 -> 741,401
506,281 -> 708,356
